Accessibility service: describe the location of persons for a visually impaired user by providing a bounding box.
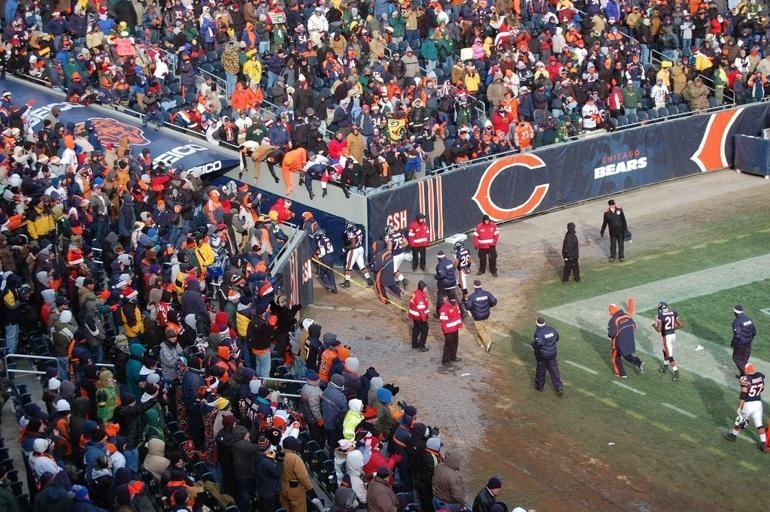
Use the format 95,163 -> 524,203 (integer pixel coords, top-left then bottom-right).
607,304 -> 645,379
0,92 -> 535,512
730,304 -> 757,379
561,222 -> 581,283
531,317 -> 563,396
723,363 -> 769,453
651,302 -> 684,381
600,200 -> 627,263
0,0 -> 770,203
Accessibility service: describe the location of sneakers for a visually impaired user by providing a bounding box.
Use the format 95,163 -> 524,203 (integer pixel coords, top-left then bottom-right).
660,368 -> 666,373
615,373 -> 628,379
339,282 -> 350,288
486,340 -> 493,352
420,347 -> 428,352
672,376 -> 681,382
442,362 -> 453,366
537,386 -> 544,391
493,273 -> 497,277
480,345 -> 486,348
723,433 -> 736,443
609,257 -> 615,263
757,442 -> 767,454
454,358 -> 461,361
412,265 -> 417,269
619,257 -> 624,262
640,361 -> 645,375
557,389 -> 563,397
736,374 -> 742,380
476,271 -> 485,275
421,266 -> 428,272
576,278 -> 582,283
368,281 -> 374,285
563,280 -> 568,285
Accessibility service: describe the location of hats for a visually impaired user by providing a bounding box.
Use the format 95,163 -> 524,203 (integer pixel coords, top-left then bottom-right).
33,438 -> 51,453
267,428 -> 282,445
566,223 -> 576,231
268,210 -> 278,221
99,368 -> 113,381
2,127 -> 11,134
608,200 -> 616,205
364,437 -> 379,448
44,119 -> 51,126
55,296 -> 70,303
259,285 -> 273,296
93,184 -> 101,190
536,318 -> 545,327
258,436 -> 271,452
231,275 -> 246,286
119,161 -> 128,169
166,329 -> 176,338
120,288 -> 138,299
258,387 -> 270,399
284,199 -> 292,206
232,426 -> 248,439
436,251 -> 445,259
79,154 -> 90,165
239,184 -> 249,191
228,290 -> 240,301
112,279 -> 126,289
221,414 -> 237,429
302,212 -> 313,222
283,436 -> 302,451
72,485 -> 88,502
256,304 -> 266,314
745,363 -> 754,373
355,431 -> 372,444
205,376 -> 219,392
474,280 -> 481,288
57,399 -> 71,411
416,214 -> 425,224
307,369 -> 320,385
734,305 -> 744,314
215,397 -> 231,411
608,304 -> 621,316
38,154 -> 49,162
240,297 -> 250,306
210,190 -> 220,200
338,439 -> 352,450
263,215 -> 272,224
51,156 -> 60,164
487,477 -> 501,489
49,377 -> 60,389
377,466 -> 393,480
71,251 -> 84,264
115,335 -> 128,345
483,215 -> 490,223
84,278 -> 97,284
1,91 -> 11,99
252,245 -> 261,251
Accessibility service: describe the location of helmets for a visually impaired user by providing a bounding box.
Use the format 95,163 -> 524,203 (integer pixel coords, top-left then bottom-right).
454,241 -> 464,253
658,302 -> 669,314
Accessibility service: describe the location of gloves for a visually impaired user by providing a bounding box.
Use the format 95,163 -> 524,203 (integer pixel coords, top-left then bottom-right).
601,233 -> 604,237
239,172 -> 243,179
736,408 -> 742,415
344,191 -> 351,198
254,178 -> 258,184
275,177 -> 280,183
309,191 -> 315,200
299,179 -> 305,186
322,188 -> 327,197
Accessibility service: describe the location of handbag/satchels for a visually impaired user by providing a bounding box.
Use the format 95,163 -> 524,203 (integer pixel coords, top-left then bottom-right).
624,231 -> 631,241
